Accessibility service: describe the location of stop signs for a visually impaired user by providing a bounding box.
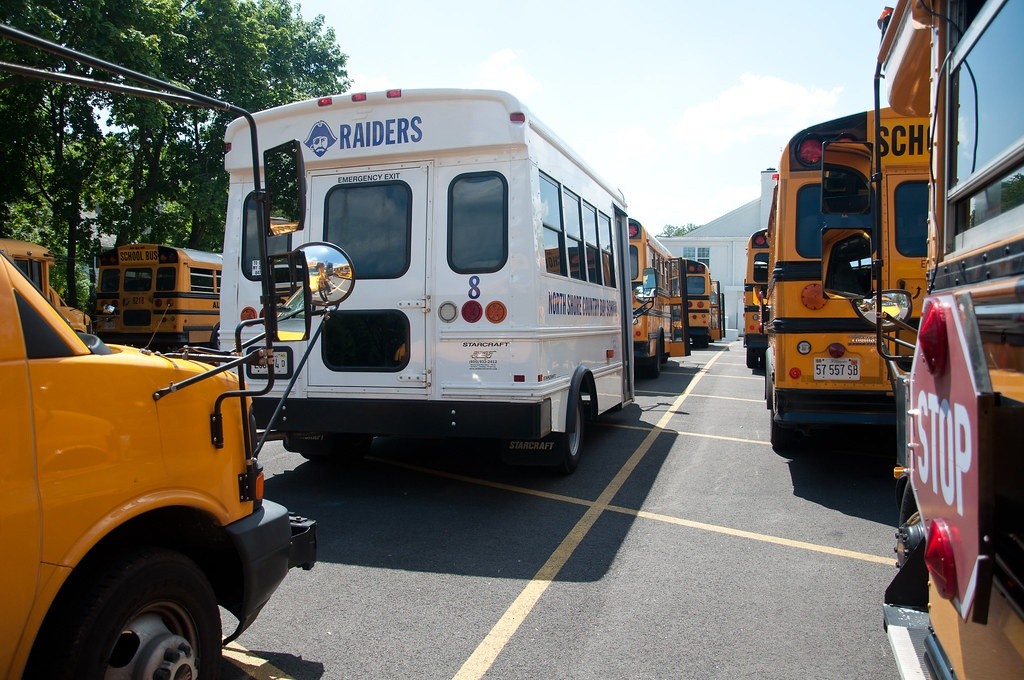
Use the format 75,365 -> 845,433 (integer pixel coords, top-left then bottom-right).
904,295 -> 996,627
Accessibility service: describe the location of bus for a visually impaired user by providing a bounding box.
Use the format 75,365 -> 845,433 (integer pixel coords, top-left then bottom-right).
742,227 -> 768,368
219,86 -> 658,480
673,257 -> 727,347
758,103 -> 932,462
0,239 -> 356,680
332,264 -> 353,280
0,236 -> 116,336
91,241 -> 221,349
868,0 -> 1024,680
307,260 -> 327,292
629,218 -> 692,379
